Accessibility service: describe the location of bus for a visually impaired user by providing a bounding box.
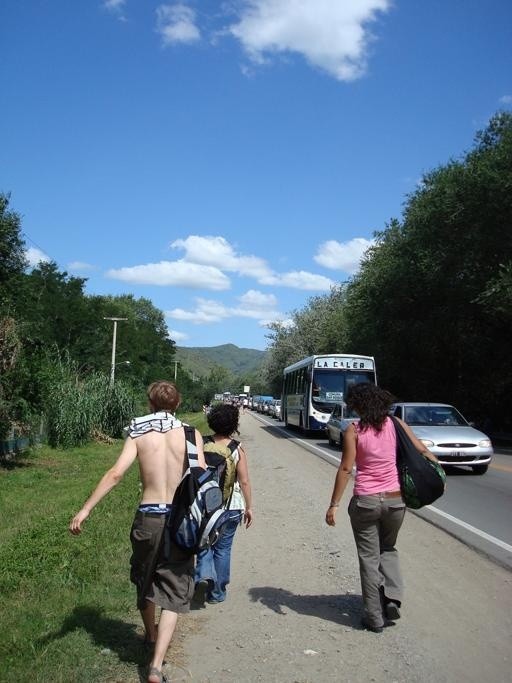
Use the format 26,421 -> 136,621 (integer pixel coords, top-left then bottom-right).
279,352 -> 380,434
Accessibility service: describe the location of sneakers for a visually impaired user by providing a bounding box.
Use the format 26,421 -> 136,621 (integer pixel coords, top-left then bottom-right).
364,600 -> 401,634
193,580 -> 219,610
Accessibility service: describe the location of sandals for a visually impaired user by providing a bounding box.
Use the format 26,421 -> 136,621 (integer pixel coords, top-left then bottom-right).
146,623 -> 161,651
144,667 -> 169,683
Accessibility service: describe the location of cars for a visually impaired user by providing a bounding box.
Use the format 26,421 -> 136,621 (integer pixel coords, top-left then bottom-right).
326,399 -> 362,448
387,401 -> 496,478
221,391 -> 282,419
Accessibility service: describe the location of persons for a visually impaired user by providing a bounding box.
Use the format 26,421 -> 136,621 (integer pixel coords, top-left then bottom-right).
242,397 -> 249,415
326,382 -> 446,633
194,403 -> 253,604
69,380 -> 230,683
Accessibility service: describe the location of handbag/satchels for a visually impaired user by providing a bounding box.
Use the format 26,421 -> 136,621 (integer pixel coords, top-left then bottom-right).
389,416 -> 447,510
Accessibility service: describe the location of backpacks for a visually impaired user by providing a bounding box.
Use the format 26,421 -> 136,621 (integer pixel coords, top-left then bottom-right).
168,425 -> 241,558
